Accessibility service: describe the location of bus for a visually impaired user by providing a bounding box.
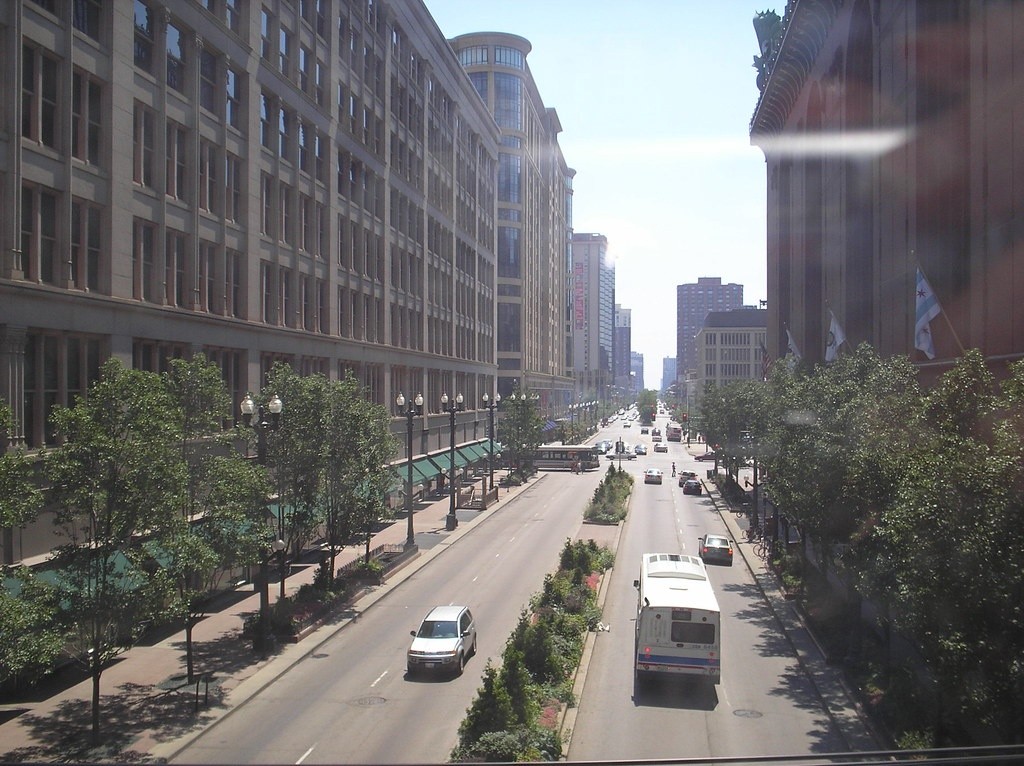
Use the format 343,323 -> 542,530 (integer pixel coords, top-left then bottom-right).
519,445 -> 602,470
633,553 -> 721,687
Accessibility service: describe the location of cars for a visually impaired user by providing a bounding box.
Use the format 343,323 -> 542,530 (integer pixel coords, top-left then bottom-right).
607,400 -> 682,442
644,468 -> 663,485
698,533 -> 733,565
683,479 -> 702,495
695,451 -> 770,468
595,439 -> 648,460
653,442 -> 668,453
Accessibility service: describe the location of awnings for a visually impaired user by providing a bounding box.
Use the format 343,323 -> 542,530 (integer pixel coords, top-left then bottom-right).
0,550 -> 150,643
541,422 -> 558,432
470,443 -> 490,459
266,469 -> 398,525
412,458 -> 440,479
481,439 -> 502,454
457,446 -> 480,464
444,450 -> 468,469
141,513 -> 278,574
431,454 -> 455,473
394,463 -> 426,486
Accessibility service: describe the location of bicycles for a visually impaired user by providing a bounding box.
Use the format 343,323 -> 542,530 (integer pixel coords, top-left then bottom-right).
736,501 -> 778,559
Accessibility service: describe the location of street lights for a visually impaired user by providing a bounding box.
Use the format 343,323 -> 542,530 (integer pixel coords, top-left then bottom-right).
240,392 -> 282,652
396,392 -> 423,545
441,392 -> 464,530
482,392 -> 501,488
510,393 -> 527,474
568,400 -> 599,445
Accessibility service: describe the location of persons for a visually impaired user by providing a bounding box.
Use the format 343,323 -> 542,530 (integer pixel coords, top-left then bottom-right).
572,460 -> 586,474
672,462 -> 676,477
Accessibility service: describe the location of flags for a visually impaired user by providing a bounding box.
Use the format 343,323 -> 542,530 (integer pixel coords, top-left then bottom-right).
825,317 -> 846,361
762,346 -> 772,367
787,330 -> 800,357
915,268 -> 940,359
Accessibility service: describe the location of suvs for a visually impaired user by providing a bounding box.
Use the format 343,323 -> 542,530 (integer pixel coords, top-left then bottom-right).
407,606 -> 477,676
678,470 -> 698,487
